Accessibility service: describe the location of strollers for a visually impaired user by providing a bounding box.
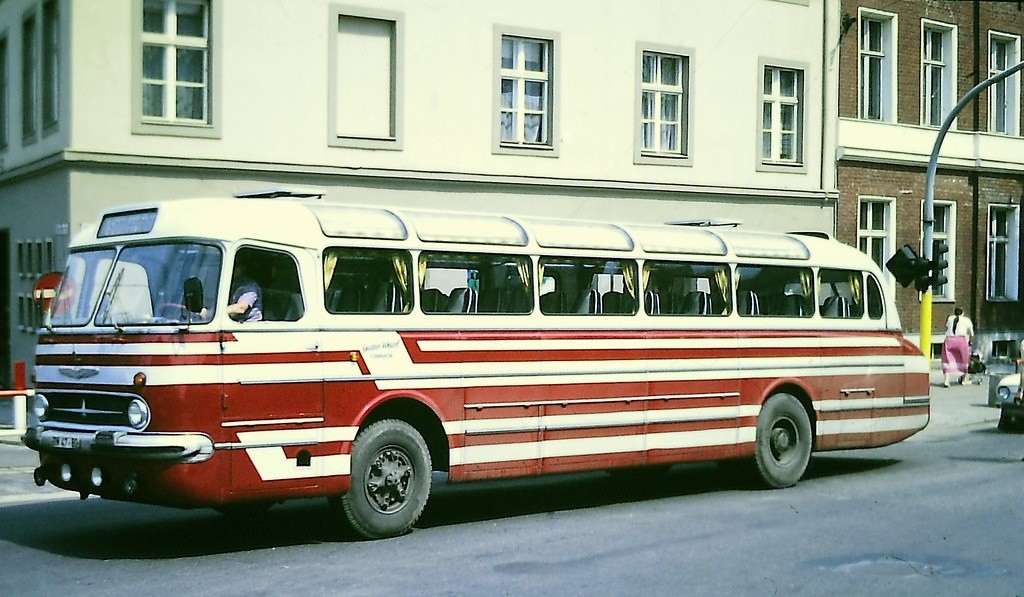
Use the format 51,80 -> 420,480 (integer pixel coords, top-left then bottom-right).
961,342 -> 986,384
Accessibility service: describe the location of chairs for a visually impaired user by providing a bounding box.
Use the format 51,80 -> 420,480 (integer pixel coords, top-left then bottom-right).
330,277 -> 852,318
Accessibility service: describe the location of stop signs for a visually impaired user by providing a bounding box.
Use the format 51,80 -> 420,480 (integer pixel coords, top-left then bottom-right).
33,272 -> 75,313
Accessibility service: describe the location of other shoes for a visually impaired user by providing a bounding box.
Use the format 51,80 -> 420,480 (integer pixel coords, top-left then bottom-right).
944,381 -> 950,387
962,380 -> 972,385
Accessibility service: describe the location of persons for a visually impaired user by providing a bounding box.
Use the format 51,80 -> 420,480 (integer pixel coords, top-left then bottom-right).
940,307 -> 984,387
201,258 -> 267,324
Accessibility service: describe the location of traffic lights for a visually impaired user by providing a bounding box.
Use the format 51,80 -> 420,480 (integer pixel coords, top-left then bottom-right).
931,241 -> 949,290
918,258 -> 933,290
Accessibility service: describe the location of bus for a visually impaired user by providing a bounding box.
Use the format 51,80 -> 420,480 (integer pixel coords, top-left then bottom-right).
23,186 -> 933,542
